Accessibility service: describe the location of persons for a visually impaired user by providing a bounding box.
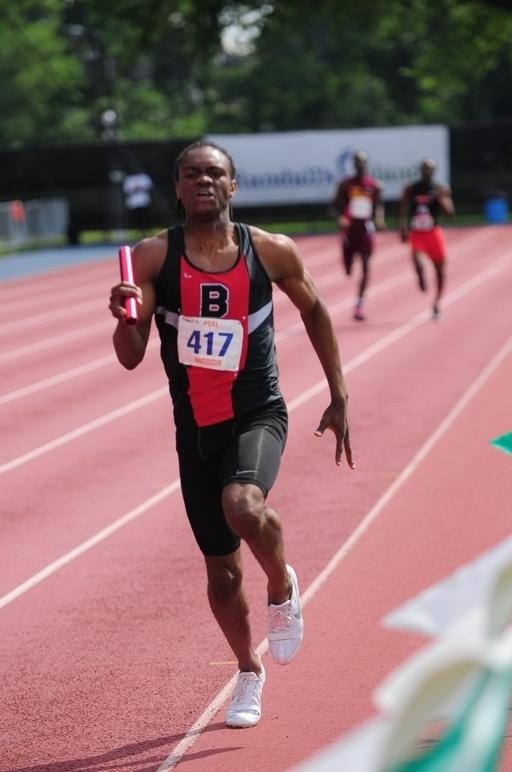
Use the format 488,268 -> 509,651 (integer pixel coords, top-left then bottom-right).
122,158 -> 156,228
108,142 -> 356,728
337,153 -> 385,320
399,159 -> 455,317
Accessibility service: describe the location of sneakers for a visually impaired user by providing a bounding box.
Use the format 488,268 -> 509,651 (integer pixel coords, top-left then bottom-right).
267,564 -> 304,666
345,260 -> 444,320
225,653 -> 266,728
